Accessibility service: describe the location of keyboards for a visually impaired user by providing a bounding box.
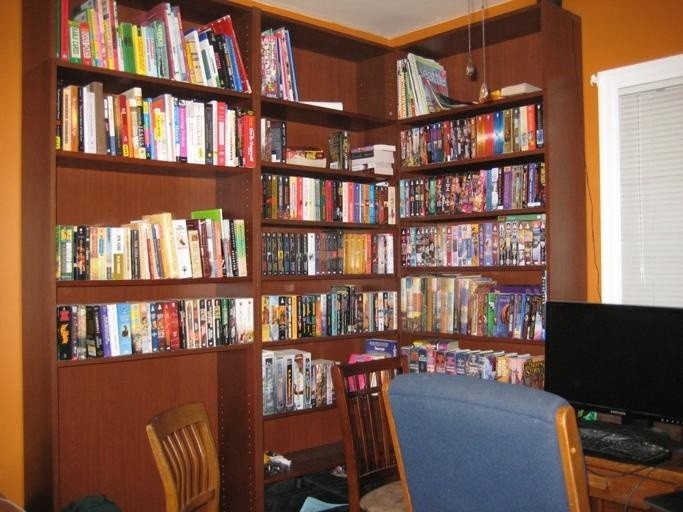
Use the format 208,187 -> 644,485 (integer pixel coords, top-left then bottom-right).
576,420 -> 671,467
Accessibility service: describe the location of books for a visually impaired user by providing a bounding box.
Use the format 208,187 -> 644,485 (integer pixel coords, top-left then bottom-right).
400,342 -> 544,390
261,118 -> 395,176
57,297 -> 254,361
54,0 -> 252,94
55,78 -> 255,169
261,173 -> 396,225
261,284 -> 399,343
402,103 -> 544,168
400,214 -> 547,267
399,163 -> 545,218
395,53 -> 544,119
262,338 -> 398,413
261,228 -> 394,277
400,272 -> 547,341
56,208 -> 248,281
259,27 -> 343,110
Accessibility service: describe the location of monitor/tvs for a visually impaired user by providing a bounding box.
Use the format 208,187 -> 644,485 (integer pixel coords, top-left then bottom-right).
544,301 -> 683,428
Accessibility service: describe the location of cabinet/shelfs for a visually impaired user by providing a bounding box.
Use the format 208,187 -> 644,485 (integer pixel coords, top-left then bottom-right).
399,1 -> 587,389
257,1 -> 399,512
21,1 -> 257,511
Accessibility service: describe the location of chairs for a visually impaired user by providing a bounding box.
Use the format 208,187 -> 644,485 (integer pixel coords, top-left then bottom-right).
331,353 -> 409,512
382,372 -> 592,512
145,402 -> 221,512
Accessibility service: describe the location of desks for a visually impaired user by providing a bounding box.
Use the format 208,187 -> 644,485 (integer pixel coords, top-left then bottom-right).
584,413 -> 682,511
